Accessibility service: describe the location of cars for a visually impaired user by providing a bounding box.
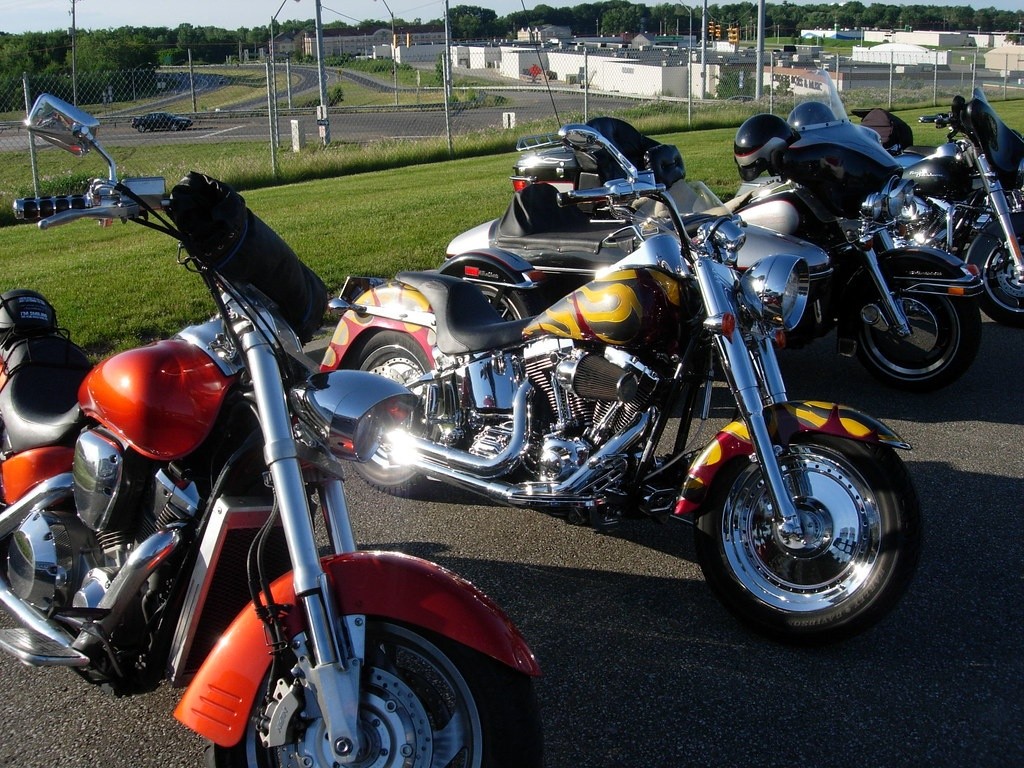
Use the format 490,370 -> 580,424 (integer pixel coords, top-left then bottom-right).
130,112 -> 194,134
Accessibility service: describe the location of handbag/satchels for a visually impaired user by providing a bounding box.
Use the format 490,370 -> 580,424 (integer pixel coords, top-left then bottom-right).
861,107 -> 913,156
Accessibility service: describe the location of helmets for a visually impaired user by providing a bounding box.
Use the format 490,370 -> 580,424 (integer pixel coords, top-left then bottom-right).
0,289 -> 57,331
734,113 -> 802,180
786,101 -> 836,129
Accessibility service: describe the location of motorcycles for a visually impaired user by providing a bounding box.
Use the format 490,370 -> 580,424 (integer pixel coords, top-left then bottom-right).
0,95 -> 547,767
309,121 -> 925,651
441,82 -> 1024,389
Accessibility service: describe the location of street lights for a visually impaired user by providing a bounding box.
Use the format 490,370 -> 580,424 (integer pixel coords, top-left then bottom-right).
131,61 -> 151,102
